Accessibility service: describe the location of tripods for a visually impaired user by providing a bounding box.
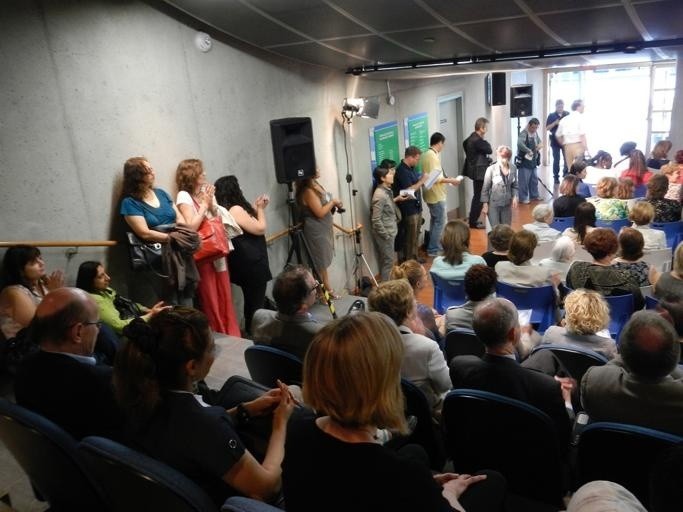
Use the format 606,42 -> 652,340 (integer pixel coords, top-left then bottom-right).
284,183 -> 337,320
345,175 -> 379,297
508,117 -> 555,198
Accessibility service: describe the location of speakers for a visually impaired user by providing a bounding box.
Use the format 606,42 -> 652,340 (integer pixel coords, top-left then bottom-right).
510,84 -> 532,118
269,116 -> 317,184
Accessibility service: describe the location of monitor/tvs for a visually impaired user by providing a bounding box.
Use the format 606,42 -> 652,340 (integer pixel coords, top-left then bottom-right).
488,72 -> 506,106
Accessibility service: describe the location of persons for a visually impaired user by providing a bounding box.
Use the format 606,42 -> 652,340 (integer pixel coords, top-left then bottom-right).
1,97 -> 683,512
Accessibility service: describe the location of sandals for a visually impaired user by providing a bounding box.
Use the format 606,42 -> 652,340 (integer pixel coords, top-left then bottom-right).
319,295 -> 328,306
329,289 -> 342,300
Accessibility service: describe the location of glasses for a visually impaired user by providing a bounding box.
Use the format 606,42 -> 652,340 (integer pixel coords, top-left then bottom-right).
87,320 -> 103,330
311,280 -> 321,291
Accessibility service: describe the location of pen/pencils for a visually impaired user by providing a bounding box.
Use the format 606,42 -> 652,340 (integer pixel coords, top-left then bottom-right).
276,379 -> 303,408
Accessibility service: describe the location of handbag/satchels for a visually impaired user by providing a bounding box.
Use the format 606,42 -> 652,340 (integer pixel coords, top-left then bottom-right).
192,216 -> 230,267
514,155 -> 523,168
113,294 -> 142,320
213,375 -> 304,447
126,224 -> 176,273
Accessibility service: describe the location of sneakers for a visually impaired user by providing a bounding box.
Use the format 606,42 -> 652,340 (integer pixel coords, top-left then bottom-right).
428,251 -> 446,256
554,178 -> 560,184
470,223 -> 484,229
530,197 -> 544,201
519,201 -> 531,204
